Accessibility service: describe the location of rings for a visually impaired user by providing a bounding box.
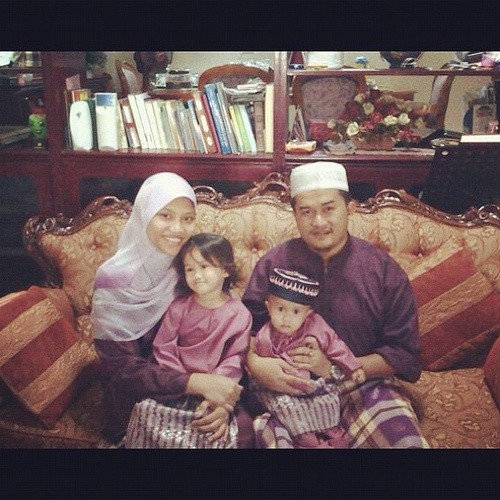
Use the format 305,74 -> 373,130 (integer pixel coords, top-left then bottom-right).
308,351 -> 313,357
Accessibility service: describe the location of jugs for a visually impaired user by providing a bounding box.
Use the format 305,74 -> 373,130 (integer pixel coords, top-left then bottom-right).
166,70 -> 192,89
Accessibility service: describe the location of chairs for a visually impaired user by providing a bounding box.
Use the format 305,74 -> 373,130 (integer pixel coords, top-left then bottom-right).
115,58 -> 142,100
200,60 -> 275,91
413,61 -> 458,130
292,67 -> 369,120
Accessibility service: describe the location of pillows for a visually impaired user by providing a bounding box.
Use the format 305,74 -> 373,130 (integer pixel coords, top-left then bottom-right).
0,285 -> 101,429
400,231 -> 500,372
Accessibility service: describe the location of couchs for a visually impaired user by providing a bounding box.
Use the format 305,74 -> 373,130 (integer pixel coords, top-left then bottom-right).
0,173 -> 500,448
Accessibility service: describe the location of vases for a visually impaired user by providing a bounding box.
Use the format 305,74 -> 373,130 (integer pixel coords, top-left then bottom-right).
350,135 -> 397,151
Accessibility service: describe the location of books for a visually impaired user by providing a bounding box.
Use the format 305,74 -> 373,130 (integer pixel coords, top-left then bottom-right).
62,78 -> 274,155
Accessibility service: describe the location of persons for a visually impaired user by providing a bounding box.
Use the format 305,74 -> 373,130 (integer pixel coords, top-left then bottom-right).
242,163 -> 430,448
253,266 -> 367,448
90,171 -> 255,449
121,232 -> 252,449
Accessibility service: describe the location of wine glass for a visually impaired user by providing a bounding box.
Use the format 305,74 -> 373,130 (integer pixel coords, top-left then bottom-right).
28,113 -> 47,150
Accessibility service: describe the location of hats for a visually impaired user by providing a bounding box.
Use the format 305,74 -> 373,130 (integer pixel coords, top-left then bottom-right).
290,161 -> 349,199
268,268 -> 321,305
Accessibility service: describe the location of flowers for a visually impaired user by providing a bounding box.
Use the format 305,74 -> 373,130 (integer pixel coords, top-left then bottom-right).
328,87 -> 425,141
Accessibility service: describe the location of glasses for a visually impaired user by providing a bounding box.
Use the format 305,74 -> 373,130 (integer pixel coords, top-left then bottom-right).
97,61 -> 106,68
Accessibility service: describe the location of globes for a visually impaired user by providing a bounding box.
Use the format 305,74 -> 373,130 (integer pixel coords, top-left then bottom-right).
380,51 -> 421,69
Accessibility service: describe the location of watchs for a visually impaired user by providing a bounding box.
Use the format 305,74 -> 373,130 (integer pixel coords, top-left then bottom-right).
330,364 -> 346,384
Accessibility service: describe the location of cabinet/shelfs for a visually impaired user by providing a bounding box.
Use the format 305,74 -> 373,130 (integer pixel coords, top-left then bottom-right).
0,51 -> 499,215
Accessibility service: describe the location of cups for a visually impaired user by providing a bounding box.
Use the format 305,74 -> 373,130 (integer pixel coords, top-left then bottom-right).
356,57 -> 368,69
156,74 -> 166,86
192,73 -> 199,85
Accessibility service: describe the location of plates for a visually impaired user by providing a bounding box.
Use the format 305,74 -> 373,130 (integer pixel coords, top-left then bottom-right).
288,141 -> 316,153
306,65 -> 328,71
322,140 -> 357,154
